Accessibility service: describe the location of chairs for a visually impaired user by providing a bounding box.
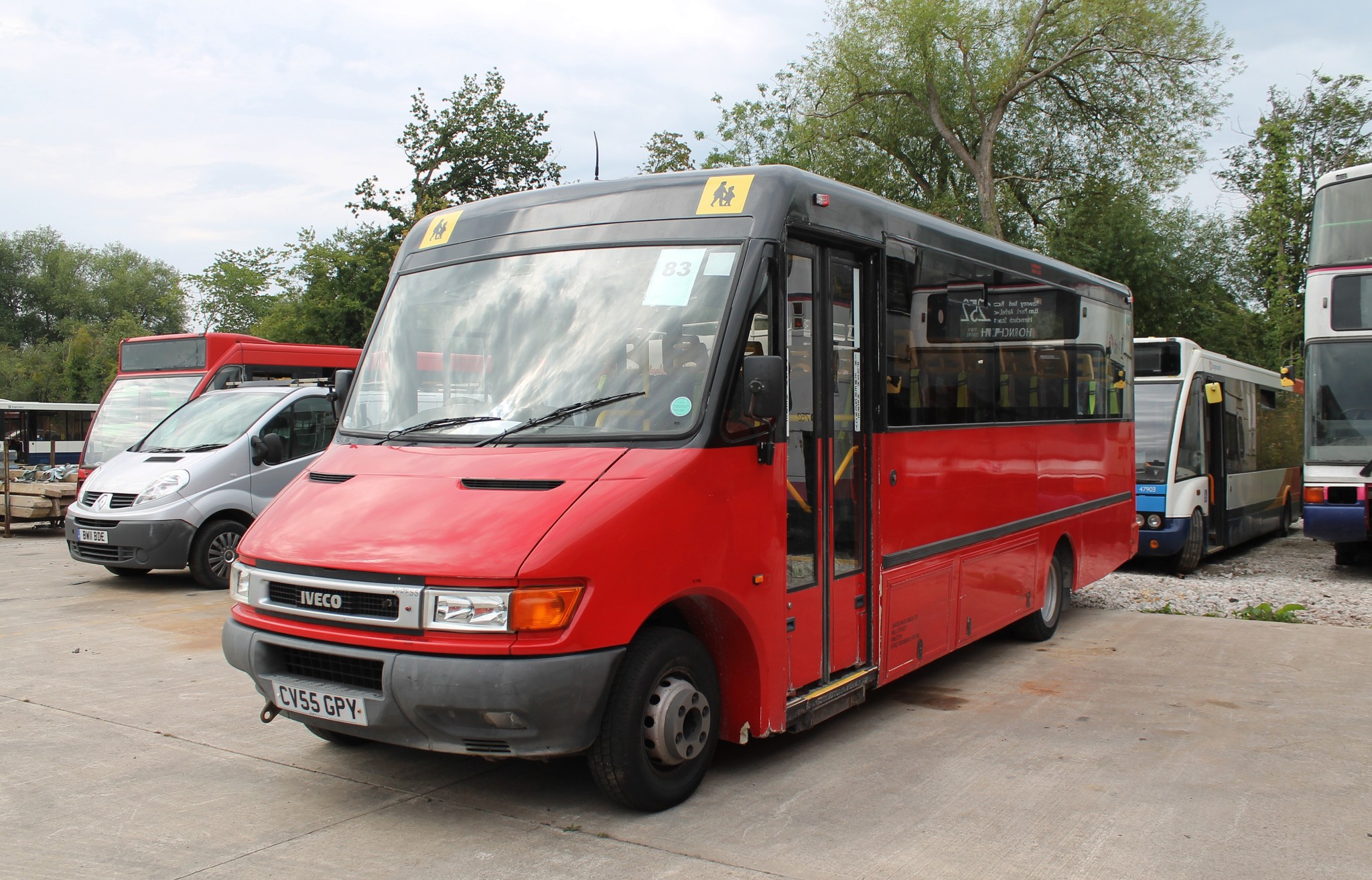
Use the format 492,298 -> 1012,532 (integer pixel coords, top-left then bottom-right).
663,337 -> 1112,497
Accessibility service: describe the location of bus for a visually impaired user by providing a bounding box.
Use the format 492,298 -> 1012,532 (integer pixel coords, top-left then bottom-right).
76,333 -> 490,517
1280,164 -> 1372,561
1,398 -> 100,468
1132,335 -> 1304,578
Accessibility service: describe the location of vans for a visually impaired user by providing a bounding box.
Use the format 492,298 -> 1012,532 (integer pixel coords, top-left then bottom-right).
236,167 -> 1139,813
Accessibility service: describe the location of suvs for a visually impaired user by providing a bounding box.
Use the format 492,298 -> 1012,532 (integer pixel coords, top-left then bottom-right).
63,384 -> 484,592
